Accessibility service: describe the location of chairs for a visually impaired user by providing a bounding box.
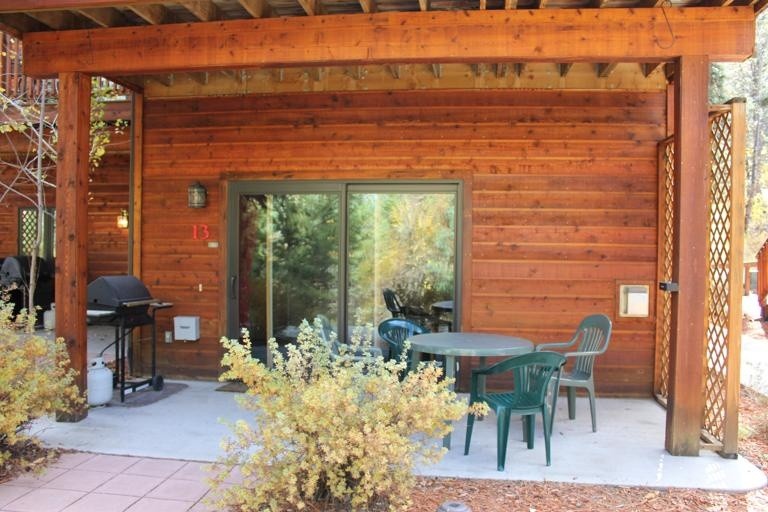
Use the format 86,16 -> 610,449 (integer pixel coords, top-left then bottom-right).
382,286 -> 456,333
377,313 -> 612,472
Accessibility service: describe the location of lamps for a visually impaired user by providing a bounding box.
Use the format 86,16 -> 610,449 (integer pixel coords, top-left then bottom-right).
186,180 -> 207,209
